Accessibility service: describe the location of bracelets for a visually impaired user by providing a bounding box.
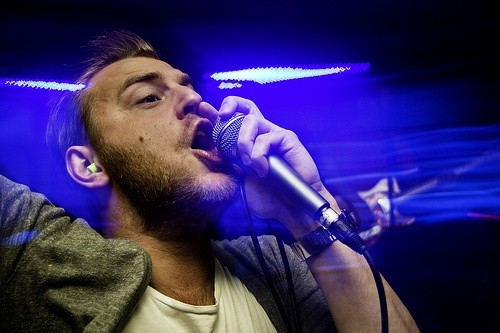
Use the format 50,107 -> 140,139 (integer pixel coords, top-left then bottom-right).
289,204 -> 359,264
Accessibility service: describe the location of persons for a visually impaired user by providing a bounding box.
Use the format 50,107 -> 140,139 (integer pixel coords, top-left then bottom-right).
2,36 -> 420,333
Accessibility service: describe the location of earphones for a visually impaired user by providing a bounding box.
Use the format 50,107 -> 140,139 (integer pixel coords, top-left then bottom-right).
87,163 -> 99,173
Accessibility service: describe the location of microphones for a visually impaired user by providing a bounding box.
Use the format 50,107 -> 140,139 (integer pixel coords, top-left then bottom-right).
213,110 -> 366,254
